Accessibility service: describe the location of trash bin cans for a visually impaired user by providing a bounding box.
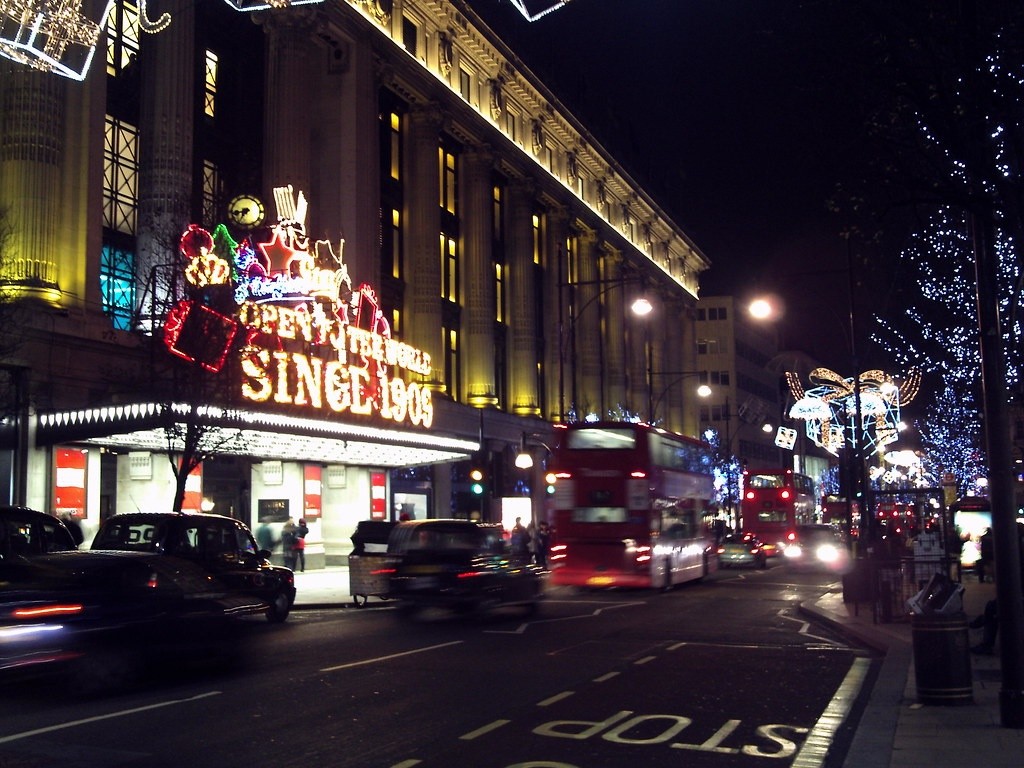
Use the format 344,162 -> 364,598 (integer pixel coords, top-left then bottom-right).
909,612 -> 974,706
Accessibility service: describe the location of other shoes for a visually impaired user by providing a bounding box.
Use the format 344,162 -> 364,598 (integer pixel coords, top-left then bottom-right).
970,643 -> 994,654
969,616 -> 987,629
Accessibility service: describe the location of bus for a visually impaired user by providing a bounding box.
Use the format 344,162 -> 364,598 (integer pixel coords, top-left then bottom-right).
547,421 -> 719,589
741,469 -> 815,545
822,494 -> 860,524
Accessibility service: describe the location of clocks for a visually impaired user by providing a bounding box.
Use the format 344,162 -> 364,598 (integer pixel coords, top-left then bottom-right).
228,193 -> 266,227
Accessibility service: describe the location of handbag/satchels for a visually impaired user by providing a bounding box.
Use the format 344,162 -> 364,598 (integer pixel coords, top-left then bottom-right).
291,537 -> 304,551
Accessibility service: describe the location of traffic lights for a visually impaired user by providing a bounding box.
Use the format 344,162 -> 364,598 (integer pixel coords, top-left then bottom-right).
470,465 -> 485,496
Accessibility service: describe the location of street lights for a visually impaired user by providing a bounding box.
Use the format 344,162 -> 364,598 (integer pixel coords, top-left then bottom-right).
648,370 -> 712,427
799,428 -> 822,474
557,276 -> 652,423
747,284 -> 867,533
467,393 -> 498,523
725,421 -> 772,528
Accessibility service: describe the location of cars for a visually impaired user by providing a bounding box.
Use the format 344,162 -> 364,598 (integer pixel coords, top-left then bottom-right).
879,503 -> 911,520
0,503 -> 273,669
717,532 -> 768,569
91,511 -> 296,622
781,524 -> 847,571
381,519 -> 549,613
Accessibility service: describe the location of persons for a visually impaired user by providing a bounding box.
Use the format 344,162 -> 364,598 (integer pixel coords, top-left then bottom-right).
281,516 -> 298,571
398,512 -> 411,522
537,521 -> 551,571
973,527 -> 995,584
255,515 -> 282,562
510,516 -> 532,564
526,520 -> 539,560
946,522 -> 971,583
53,511 -> 85,550
289,518 -> 309,572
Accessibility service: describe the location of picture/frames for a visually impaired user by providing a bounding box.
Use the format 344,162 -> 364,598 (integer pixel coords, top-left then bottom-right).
257,499 -> 290,524
